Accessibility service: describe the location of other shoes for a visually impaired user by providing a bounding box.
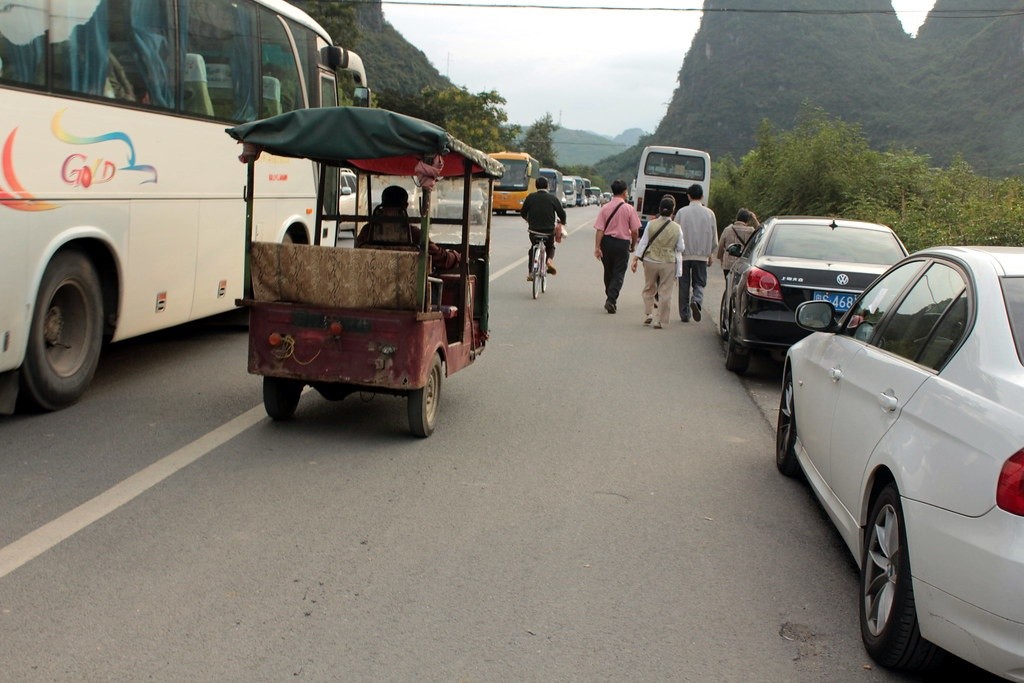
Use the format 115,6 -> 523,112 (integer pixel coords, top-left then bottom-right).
690,302 -> 701,322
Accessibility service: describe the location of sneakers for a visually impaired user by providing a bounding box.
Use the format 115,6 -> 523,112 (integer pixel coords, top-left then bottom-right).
604,301 -> 617,314
643,315 -> 653,324
654,322 -> 661,328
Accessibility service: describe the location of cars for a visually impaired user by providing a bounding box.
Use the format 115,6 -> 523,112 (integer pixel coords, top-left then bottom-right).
773,242 -> 1024,683
717,212 -> 914,378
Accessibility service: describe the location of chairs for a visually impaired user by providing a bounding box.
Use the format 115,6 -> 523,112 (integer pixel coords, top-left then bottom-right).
184,54 -> 282,118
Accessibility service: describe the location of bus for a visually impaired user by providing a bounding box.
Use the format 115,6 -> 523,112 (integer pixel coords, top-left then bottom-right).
487,151 -> 540,216
629,144 -> 712,248
0,0 -> 372,419
538,167 -> 563,209
562,175 -> 578,208
569,175 -> 614,207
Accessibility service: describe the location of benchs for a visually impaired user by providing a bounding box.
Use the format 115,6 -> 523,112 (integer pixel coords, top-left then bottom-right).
252,240 -> 458,347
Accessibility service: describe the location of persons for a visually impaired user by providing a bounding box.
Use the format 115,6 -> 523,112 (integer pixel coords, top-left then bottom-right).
357,186 -> 462,305
674,184 -> 718,322
718,209 -> 762,279
520,177 -> 566,279
632,195 -> 685,328
594,180 -> 642,314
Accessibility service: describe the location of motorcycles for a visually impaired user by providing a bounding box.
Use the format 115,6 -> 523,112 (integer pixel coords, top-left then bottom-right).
223,105 -> 507,441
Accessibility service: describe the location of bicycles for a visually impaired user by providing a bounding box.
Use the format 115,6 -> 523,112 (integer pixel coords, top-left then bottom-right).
525,218 -> 567,299
